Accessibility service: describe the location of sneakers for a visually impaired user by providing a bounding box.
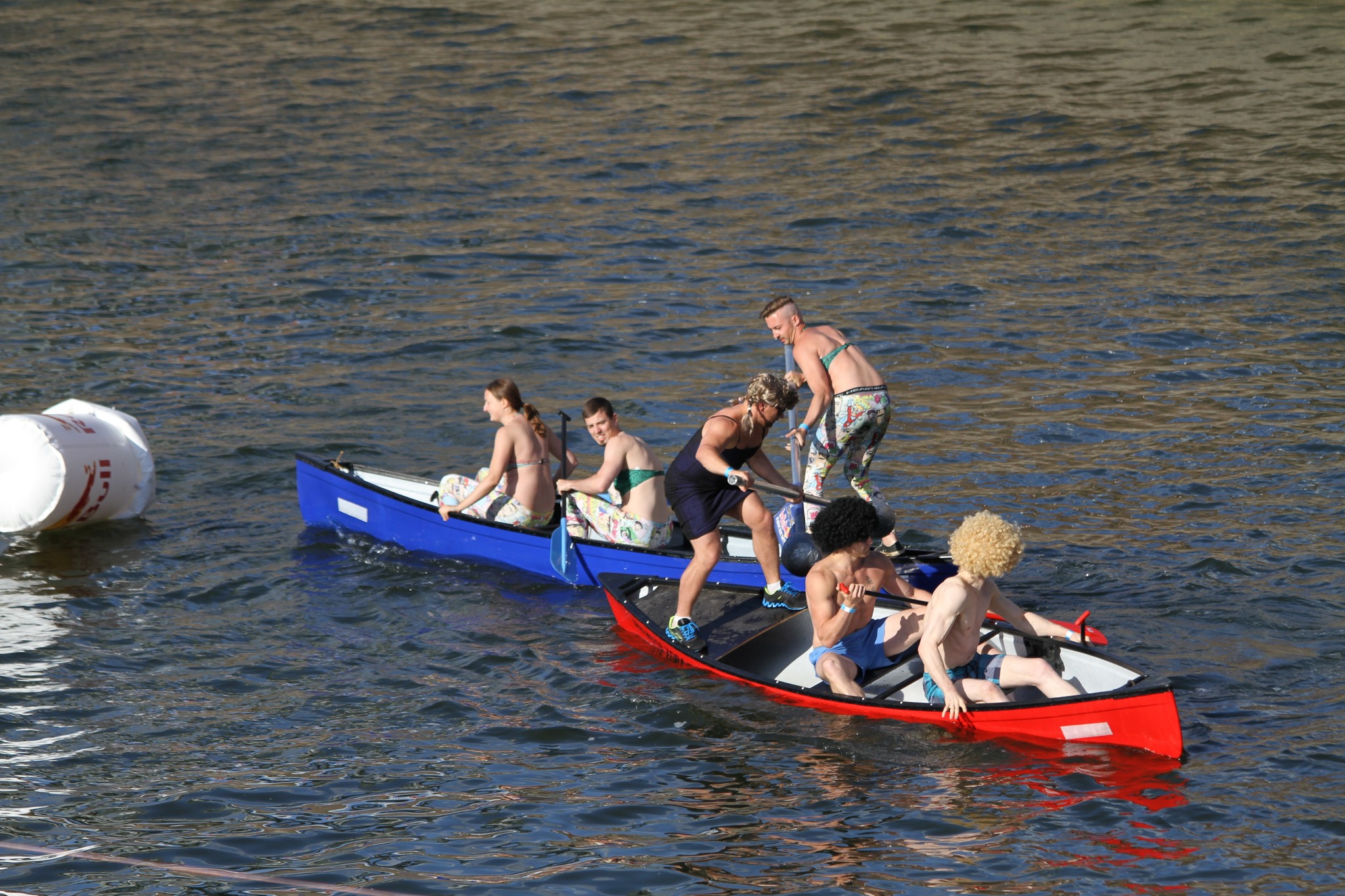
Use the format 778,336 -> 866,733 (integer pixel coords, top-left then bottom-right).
762,579 -> 808,610
873,540 -> 905,557
664,615 -> 706,653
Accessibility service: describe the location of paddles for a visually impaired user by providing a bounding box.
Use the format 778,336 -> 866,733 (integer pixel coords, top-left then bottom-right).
555,494 -> 612,504
839,581 -> 1107,645
550,409 -> 578,583
1074,610 -> 1090,645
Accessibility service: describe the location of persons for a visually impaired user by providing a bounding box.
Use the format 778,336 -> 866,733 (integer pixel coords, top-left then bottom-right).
918,509 -> 1093,720
806,495 -> 1006,696
556,397 -> 674,548
664,373 -> 808,654
759,296 -> 908,558
437,377 -> 578,531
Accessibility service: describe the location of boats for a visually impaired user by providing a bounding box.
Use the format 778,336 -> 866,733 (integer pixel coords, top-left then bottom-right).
594,570 -> 1190,762
294,450 -> 960,604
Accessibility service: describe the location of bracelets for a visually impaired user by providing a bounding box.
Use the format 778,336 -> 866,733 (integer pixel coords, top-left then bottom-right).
1065,630 -> 1074,640
799,423 -> 810,434
724,466 -> 735,478
841,604 -> 856,613
